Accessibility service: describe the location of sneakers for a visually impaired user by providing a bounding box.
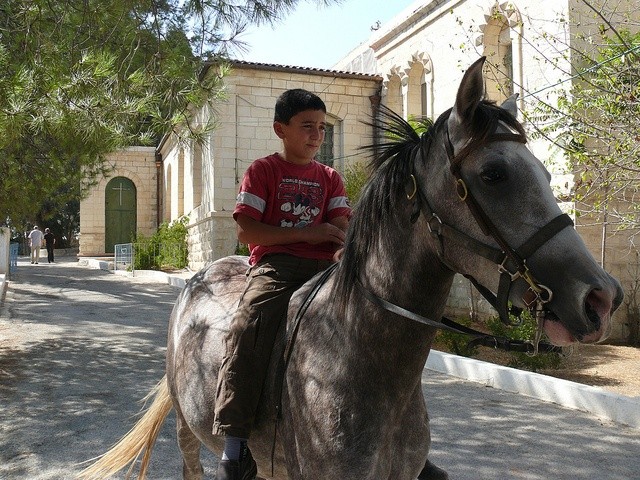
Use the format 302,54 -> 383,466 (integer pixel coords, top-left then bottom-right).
417,459 -> 450,480
31,261 -> 34,264
214,441 -> 258,480
35,262 -> 38,264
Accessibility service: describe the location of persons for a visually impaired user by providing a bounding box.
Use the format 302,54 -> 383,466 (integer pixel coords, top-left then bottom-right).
42,227 -> 57,262
211,88 -> 352,479
27,225 -> 44,264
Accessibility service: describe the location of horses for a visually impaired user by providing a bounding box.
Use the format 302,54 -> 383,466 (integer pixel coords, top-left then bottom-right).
68,56 -> 624,480
